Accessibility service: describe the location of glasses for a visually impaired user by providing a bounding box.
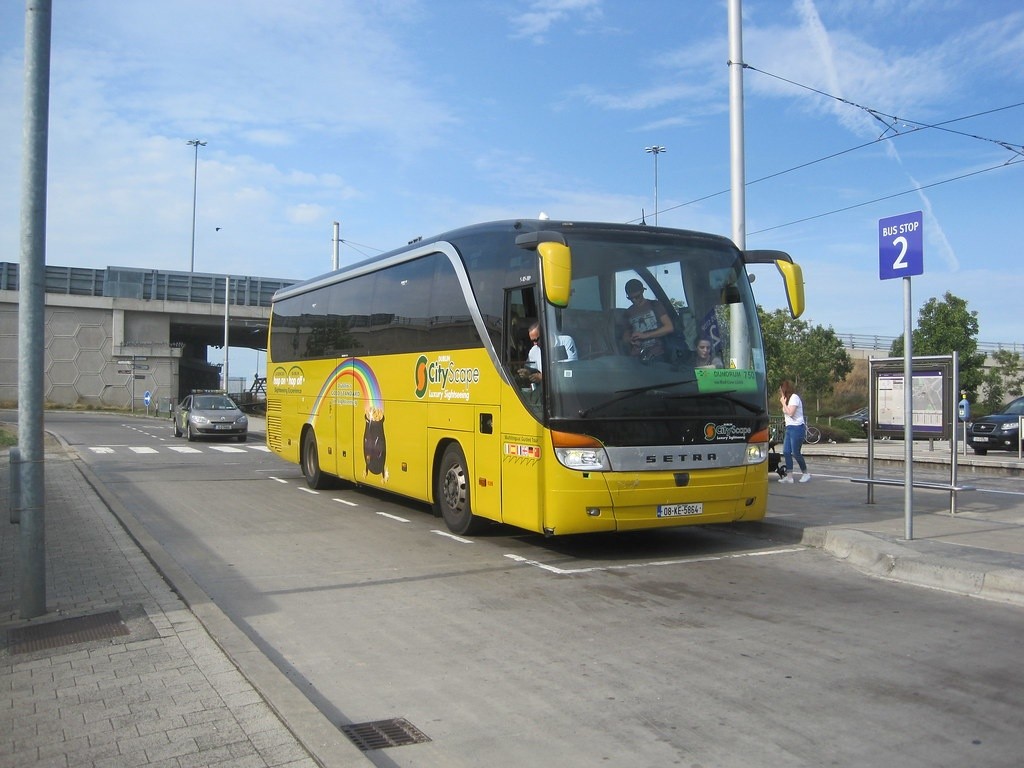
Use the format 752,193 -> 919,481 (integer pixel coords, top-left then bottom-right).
530,336 -> 541,342
626,292 -> 641,300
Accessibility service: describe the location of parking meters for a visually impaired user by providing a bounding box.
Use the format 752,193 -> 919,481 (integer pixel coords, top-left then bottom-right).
958,393 -> 969,458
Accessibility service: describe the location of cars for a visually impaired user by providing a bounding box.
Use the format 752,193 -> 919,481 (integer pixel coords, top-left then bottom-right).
967,397 -> 1024,454
173,389 -> 248,442
839,409 -> 929,441
834,406 -> 869,422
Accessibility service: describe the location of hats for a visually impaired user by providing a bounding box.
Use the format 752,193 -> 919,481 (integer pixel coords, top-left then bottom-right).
625,279 -> 647,292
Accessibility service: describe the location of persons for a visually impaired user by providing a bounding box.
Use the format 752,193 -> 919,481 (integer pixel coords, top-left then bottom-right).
777,378 -> 813,484
622,278 -> 674,356
679,338 -> 724,371
516,322 -> 579,391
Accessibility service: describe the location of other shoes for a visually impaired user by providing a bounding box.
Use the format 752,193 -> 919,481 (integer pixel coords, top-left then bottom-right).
778,477 -> 793,484
799,473 -> 810,482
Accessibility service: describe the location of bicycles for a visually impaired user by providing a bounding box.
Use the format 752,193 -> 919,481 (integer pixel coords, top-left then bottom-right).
801,421 -> 823,445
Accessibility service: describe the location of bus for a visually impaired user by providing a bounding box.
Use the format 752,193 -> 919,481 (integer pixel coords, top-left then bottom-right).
263,218 -> 806,537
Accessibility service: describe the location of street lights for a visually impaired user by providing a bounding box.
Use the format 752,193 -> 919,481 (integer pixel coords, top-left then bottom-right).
645,146 -> 666,226
186,138 -> 209,271
